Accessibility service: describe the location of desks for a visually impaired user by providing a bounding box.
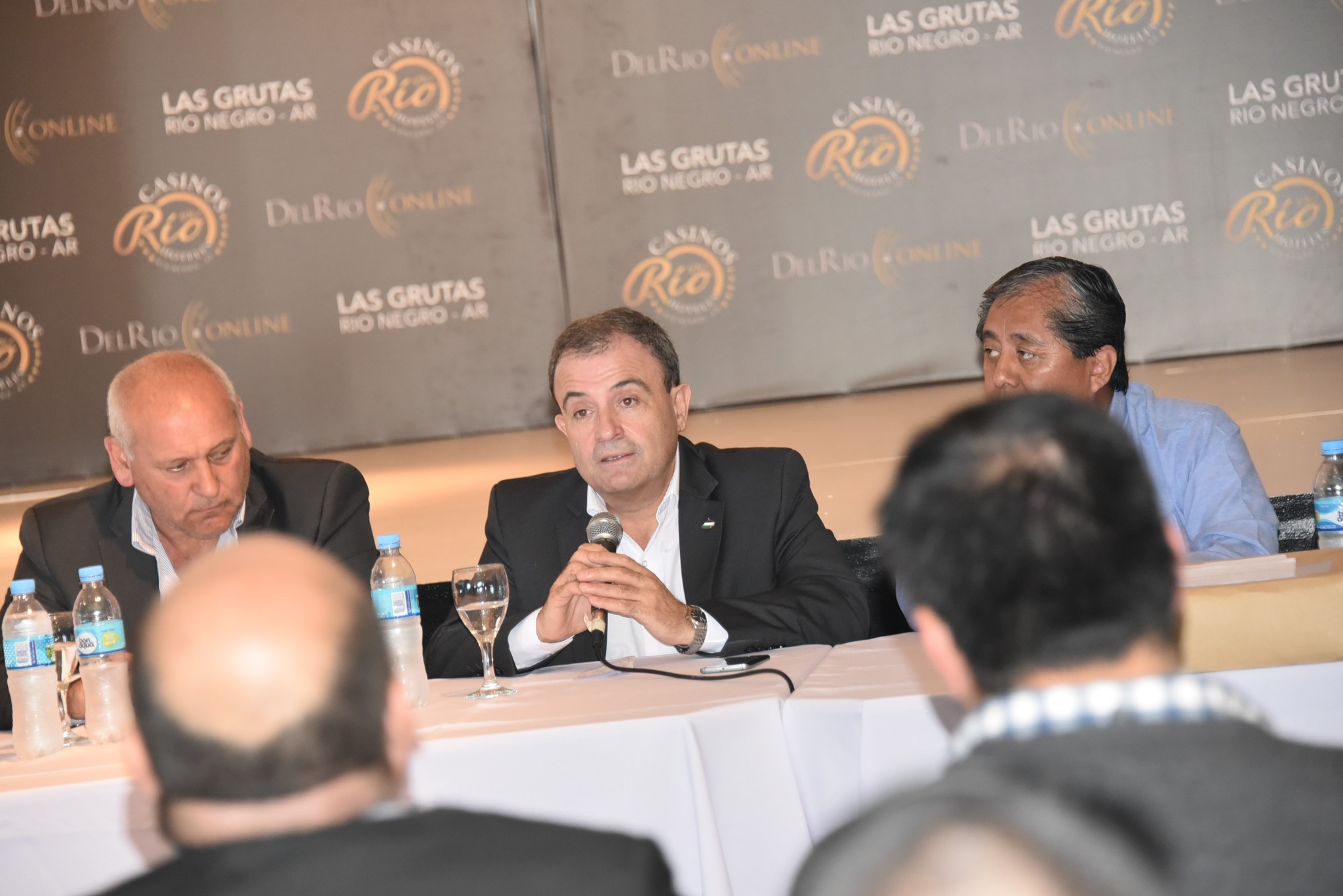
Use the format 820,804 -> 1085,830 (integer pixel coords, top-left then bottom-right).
1,623 -> 834,895
793,570 -> 1343,895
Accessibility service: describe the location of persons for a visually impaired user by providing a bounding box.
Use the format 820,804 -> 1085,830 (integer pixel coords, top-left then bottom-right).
974,254 -> 1280,563
425,306 -> 873,679
97,529 -> 674,896
789,387 -> 1343,896
0,346 -> 383,736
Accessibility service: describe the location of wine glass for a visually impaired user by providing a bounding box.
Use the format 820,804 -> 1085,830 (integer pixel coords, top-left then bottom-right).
49,610 -> 88,745
451,562 -> 517,699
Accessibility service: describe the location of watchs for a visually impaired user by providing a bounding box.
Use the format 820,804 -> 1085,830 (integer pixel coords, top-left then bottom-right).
674,605 -> 708,655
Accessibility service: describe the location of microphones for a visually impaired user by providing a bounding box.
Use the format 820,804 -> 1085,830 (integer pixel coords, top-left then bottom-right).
586,512 -> 623,649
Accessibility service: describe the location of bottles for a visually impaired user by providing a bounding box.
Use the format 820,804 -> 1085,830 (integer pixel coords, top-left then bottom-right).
370,534 -> 429,710
1313,440 -> 1343,549
0,579 -> 64,760
72,564 -> 136,749
